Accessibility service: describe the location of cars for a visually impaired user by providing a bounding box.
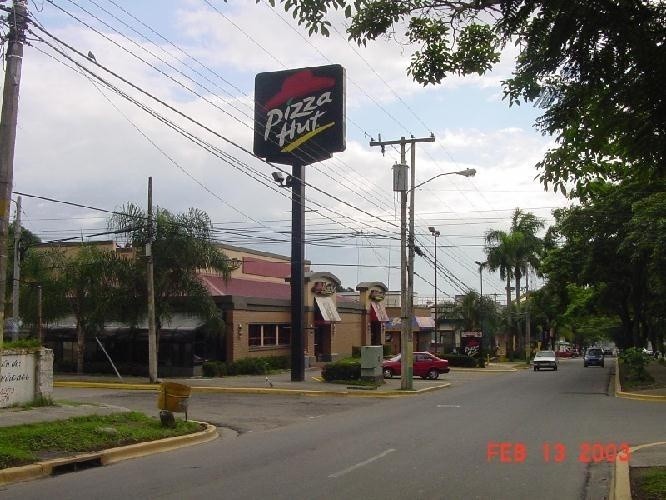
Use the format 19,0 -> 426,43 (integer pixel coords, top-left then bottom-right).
382,350 -> 451,381
556,344 -> 616,368
640,348 -> 662,359
532,350 -> 557,372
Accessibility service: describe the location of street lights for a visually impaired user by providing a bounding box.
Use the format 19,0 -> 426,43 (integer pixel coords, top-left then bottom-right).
270,171 -> 304,383
397,167 -> 478,391
474,259 -> 490,297
428,226 -> 443,356
